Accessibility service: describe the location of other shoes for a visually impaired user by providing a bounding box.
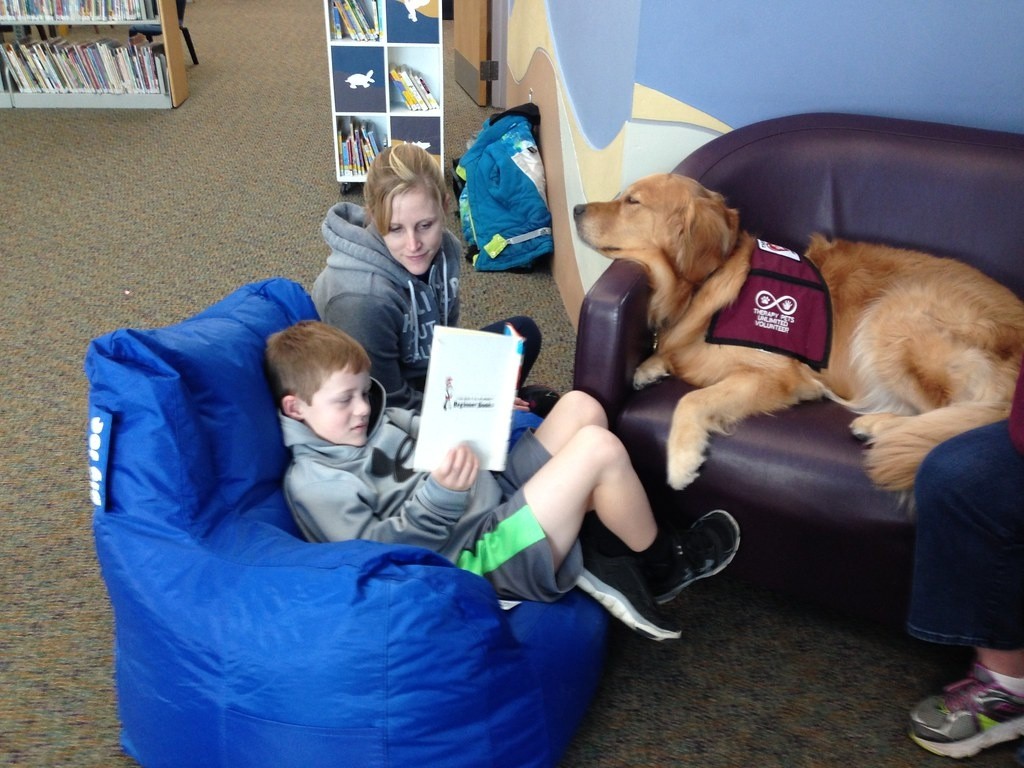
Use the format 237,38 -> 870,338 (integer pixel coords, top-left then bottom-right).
517,383 -> 562,420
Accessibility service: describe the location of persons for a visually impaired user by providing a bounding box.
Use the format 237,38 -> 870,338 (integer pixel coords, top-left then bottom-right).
311,139 -> 560,420
129,0 -> 186,45
264,323 -> 741,605
906,359 -> 1024,758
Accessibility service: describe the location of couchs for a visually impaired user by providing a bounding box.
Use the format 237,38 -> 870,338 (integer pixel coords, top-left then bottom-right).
574,113 -> 1024,602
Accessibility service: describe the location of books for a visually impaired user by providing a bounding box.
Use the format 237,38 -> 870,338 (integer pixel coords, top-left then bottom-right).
0,37 -> 170,94
390,63 -> 439,111
0,0 -> 157,22
412,323 -> 526,472
337,116 -> 388,176
331,0 -> 379,42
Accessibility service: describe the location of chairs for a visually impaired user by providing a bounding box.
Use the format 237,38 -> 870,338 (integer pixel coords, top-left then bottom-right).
86,275 -> 607,768
128,0 -> 199,66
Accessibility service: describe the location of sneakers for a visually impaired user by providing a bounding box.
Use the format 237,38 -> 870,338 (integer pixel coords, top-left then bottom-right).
573,537 -> 682,642
639,510 -> 742,605
907,661 -> 1024,759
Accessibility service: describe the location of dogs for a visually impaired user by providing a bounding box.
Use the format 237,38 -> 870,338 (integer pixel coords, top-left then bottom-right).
573,175 -> 1024,515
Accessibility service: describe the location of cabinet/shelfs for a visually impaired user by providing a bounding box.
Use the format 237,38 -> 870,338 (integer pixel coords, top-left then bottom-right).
0,0 -> 190,111
324,0 -> 446,194
